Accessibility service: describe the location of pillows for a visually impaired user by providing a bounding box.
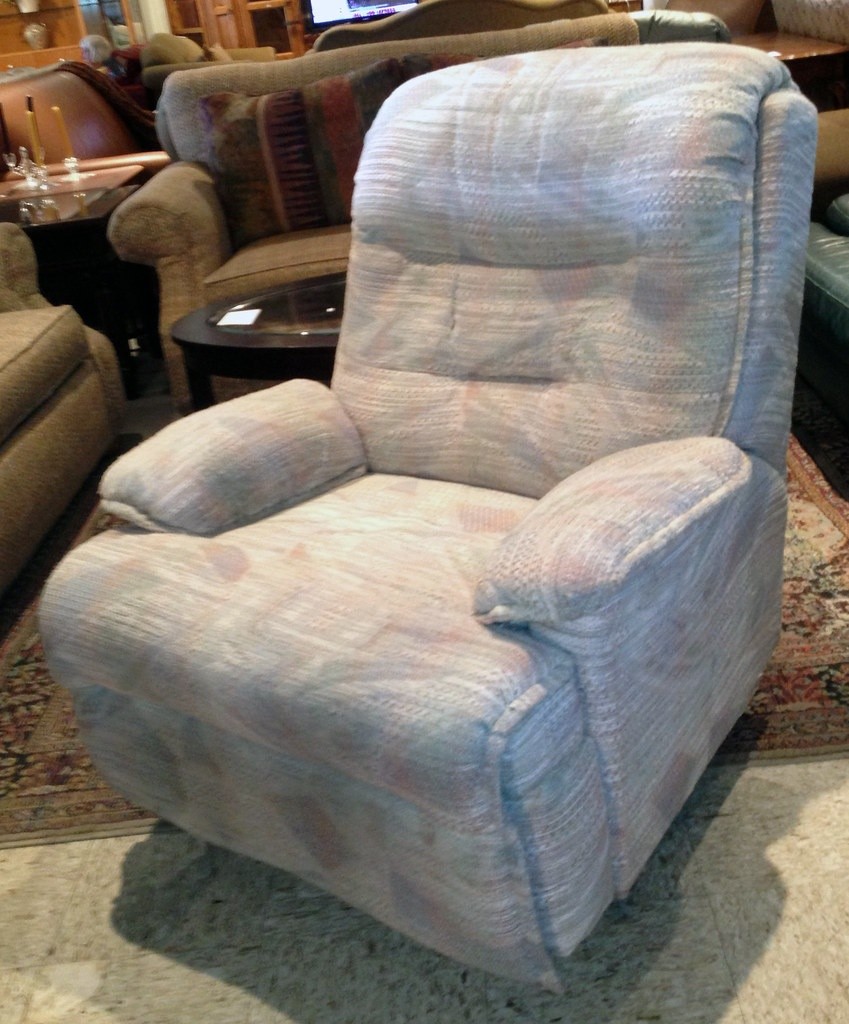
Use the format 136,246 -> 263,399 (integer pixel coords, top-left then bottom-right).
202,40 -> 233,62
405,37 -> 608,81
198,56 -> 404,249
828,193 -> 849,235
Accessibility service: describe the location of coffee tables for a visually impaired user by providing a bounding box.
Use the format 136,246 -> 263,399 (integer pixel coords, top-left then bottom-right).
170,270 -> 347,411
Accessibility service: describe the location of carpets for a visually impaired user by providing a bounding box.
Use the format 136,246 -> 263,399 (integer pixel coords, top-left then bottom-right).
0,365 -> 849,850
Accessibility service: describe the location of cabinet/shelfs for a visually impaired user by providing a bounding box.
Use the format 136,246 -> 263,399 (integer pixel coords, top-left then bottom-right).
0,0 -> 88,72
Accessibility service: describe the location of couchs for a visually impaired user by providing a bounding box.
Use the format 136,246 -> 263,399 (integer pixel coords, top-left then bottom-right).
36,39 -> 818,994
0,0 -> 849,593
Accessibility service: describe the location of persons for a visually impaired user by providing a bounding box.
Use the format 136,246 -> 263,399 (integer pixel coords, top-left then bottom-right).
79,34 -> 126,80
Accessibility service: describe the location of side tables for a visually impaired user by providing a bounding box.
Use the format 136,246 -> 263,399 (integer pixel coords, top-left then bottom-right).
0,164 -> 161,400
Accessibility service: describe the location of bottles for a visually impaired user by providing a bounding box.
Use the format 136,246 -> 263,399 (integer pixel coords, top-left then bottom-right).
23,95 -> 45,164
14,147 -> 36,178
22,21 -> 49,49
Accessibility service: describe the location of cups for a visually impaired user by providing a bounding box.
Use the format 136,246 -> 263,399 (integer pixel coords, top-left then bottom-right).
26,166 -> 48,190
4,152 -> 16,173
64,157 -> 81,180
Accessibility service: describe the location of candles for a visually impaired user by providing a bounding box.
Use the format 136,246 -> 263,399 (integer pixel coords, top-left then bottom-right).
25,96 -> 41,148
50,106 -> 73,159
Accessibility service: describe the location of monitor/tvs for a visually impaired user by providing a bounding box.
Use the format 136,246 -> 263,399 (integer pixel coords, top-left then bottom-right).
302,0 -> 419,33
